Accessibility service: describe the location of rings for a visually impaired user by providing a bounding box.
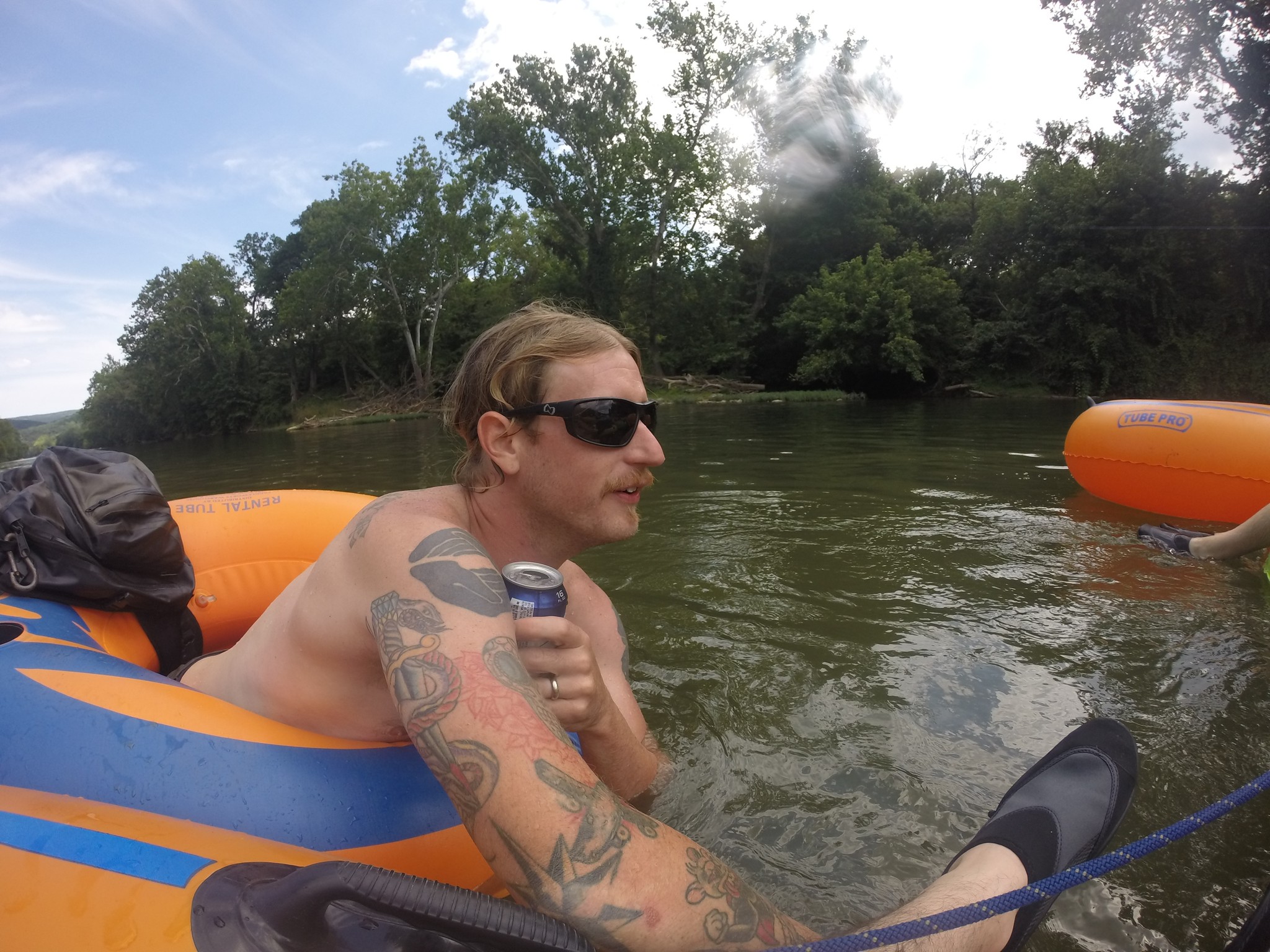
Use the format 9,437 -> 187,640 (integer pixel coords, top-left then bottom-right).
548,675 -> 559,701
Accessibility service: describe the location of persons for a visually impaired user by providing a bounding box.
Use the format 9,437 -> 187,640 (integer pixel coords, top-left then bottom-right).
849,717 -> 1139,952
170,307 -> 825,952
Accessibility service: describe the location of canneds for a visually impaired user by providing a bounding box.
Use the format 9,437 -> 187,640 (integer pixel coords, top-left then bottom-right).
501,561 -> 567,648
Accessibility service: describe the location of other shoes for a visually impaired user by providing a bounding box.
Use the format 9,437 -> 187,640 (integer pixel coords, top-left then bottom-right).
1136,519 -> 1214,559
938,718 -> 1140,952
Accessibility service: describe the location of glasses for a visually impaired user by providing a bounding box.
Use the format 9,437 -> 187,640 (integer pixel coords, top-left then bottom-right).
467,396 -> 661,448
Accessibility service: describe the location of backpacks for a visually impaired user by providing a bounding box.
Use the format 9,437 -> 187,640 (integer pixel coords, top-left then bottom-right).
0,445 -> 196,616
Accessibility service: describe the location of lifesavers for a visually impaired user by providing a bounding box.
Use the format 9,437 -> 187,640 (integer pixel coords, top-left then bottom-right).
0,486 -> 513,952
1061,398 -> 1270,527
